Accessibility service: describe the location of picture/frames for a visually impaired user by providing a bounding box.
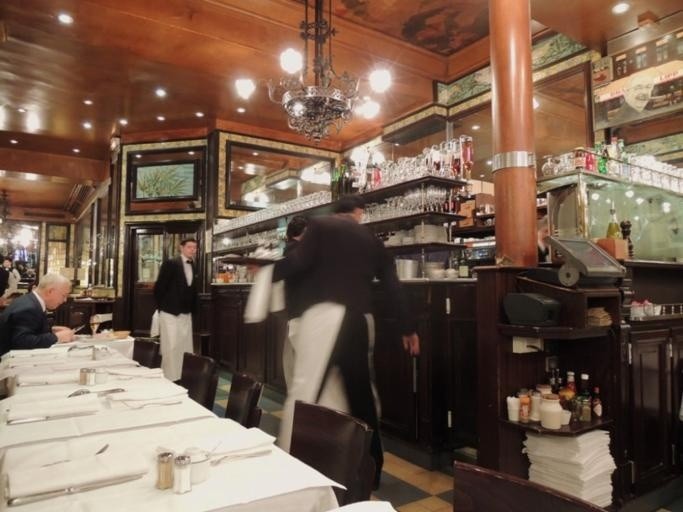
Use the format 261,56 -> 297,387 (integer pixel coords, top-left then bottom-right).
129,158 -> 201,203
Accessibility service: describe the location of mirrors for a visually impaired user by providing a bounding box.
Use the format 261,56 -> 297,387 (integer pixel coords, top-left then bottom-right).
225,139 -> 336,212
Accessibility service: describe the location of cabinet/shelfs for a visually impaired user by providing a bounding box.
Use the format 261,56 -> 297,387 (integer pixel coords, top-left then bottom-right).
498,323 -> 621,511
627,327 -> 683,490
210,175 -> 470,280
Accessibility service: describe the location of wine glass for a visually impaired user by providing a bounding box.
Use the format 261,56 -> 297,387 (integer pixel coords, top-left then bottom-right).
363,184 -> 446,223
541,154 -> 572,177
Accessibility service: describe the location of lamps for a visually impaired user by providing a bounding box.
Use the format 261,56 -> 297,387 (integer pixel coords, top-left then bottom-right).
233,1 -> 393,143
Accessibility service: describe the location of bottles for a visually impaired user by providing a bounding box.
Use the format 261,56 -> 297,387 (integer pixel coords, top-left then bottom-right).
88,369 -> 95,385
79,368 -> 88,384
606,209 -> 621,239
155,452 -> 172,489
506,368 -> 602,430
221,267 -> 239,283
574,136 -> 628,176
329,134 -> 473,202
445,186 -> 473,212
448,247 -> 494,278
173,456 -> 190,493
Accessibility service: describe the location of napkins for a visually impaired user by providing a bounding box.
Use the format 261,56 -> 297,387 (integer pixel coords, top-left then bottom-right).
4,445 -> 144,504
106,379 -> 189,409
153,426 -> 277,462
6,392 -> 102,424
105,366 -> 164,379
15,368 -> 83,387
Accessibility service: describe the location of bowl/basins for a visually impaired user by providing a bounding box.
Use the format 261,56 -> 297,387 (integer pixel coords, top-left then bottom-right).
113,331 -> 129,338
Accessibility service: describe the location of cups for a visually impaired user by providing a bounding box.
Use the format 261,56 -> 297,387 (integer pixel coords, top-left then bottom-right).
212,190 -> 331,237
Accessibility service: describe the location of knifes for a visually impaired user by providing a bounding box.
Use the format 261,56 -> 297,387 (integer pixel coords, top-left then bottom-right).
6,473 -> 142,507
7,410 -> 96,426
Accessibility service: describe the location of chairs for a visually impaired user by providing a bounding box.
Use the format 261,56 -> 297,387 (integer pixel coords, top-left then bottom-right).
170,352 -> 219,411
451,460 -> 605,511
132,337 -> 164,369
286,400 -> 375,510
221,368 -> 263,428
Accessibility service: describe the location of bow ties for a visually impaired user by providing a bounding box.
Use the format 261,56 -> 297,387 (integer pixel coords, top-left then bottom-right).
186,260 -> 193,264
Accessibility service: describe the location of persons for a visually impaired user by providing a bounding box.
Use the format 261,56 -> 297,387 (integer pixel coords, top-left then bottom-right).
281,215 -> 309,295
241,193 -> 421,491
153,238 -> 199,385
0,271 -> 75,357
2,256 -> 21,289
0,253 -> 9,297
